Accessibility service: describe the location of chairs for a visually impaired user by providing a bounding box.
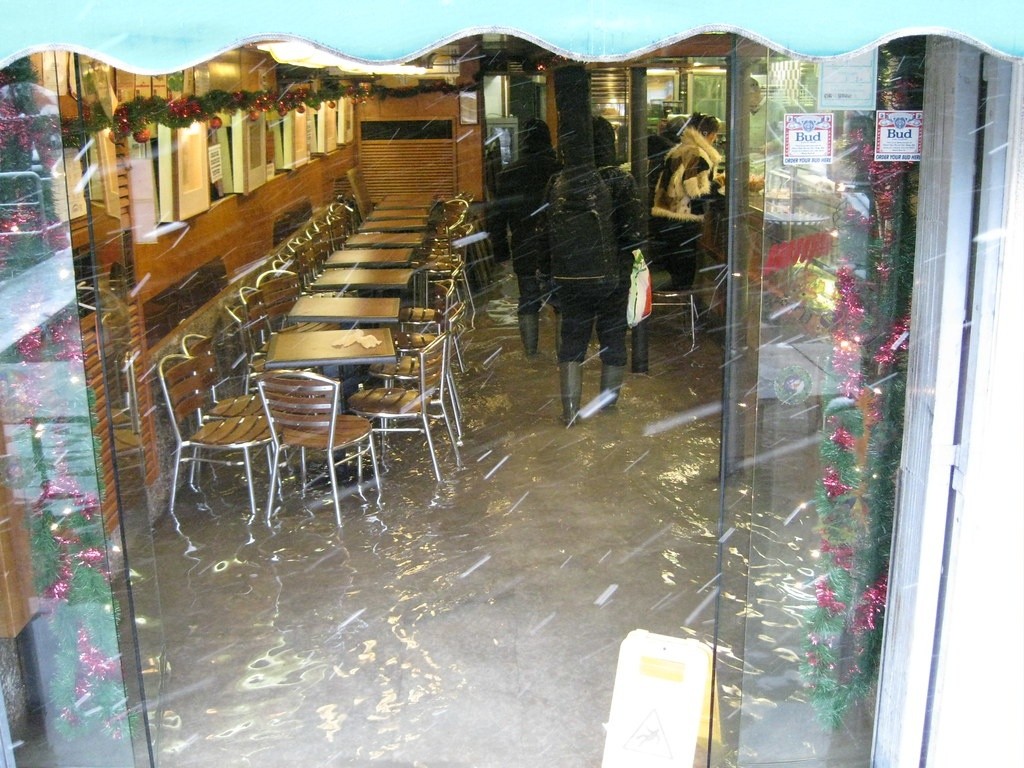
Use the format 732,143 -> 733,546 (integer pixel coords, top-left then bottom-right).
158,187 -> 382,527
348,194 -> 487,484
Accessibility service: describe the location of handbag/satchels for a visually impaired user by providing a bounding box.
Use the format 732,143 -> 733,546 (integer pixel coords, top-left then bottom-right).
626,248 -> 652,329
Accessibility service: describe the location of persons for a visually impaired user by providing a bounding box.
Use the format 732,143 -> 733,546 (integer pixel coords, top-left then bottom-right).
642,115 -> 694,263
651,111 -> 725,309
538,114 -> 648,429
497,116 -> 569,362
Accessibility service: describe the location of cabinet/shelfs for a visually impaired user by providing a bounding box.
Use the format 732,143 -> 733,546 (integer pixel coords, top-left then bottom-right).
694,153 -> 871,351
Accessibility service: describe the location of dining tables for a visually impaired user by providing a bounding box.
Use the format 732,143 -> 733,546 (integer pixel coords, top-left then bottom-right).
264,190 -> 438,417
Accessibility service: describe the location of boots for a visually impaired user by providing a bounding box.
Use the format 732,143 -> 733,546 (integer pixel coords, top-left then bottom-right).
598,363 -> 625,412
517,313 -> 539,356
556,313 -> 562,360
558,362 -> 582,427
